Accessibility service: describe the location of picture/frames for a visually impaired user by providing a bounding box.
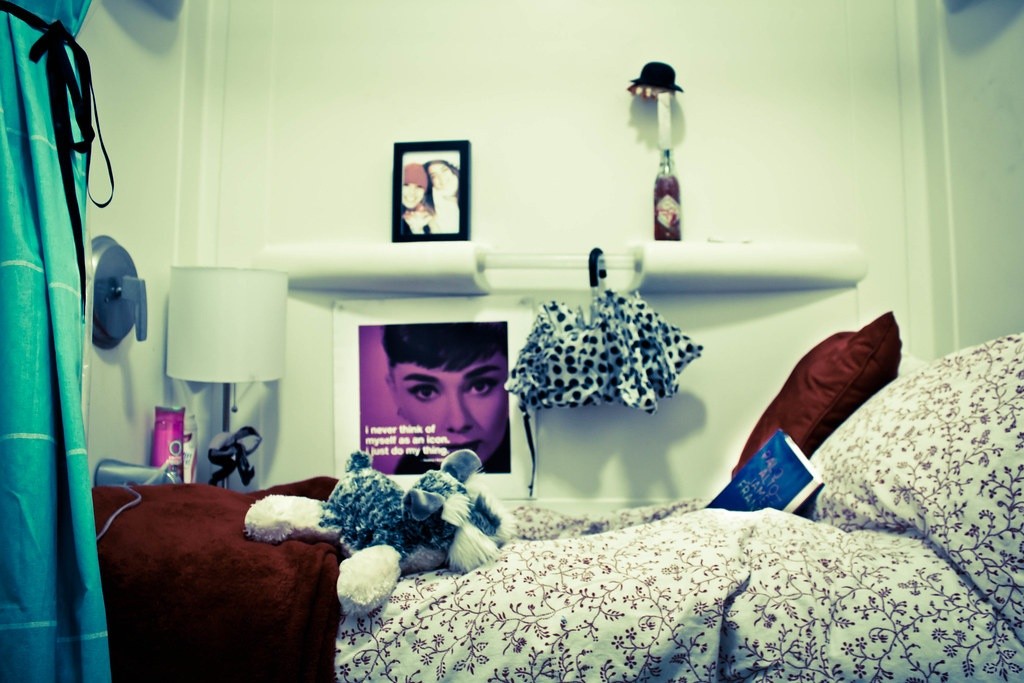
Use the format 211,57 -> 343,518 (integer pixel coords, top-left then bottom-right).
390,141 -> 471,243
329,293 -> 541,503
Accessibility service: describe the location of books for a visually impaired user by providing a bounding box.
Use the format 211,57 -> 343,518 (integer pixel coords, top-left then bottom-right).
704,428 -> 826,513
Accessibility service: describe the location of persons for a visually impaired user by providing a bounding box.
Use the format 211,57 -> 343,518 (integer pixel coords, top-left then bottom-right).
382,323 -> 510,474
425,159 -> 461,234
402,164 -> 433,234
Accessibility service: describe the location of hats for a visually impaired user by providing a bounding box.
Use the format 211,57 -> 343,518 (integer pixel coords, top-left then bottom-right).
402,164 -> 429,192
630,63 -> 683,98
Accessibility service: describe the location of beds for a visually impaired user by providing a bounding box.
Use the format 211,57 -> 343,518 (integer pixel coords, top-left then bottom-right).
92,332 -> 1023,683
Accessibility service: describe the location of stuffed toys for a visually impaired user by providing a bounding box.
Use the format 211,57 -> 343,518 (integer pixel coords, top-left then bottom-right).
244,445 -> 501,617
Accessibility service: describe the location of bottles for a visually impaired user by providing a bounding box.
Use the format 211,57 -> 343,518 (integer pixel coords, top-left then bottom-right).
654,149 -> 683,241
151,406 -> 184,484
183,413 -> 199,484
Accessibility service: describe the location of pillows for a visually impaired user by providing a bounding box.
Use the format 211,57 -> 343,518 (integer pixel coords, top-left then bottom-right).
731,309 -> 904,482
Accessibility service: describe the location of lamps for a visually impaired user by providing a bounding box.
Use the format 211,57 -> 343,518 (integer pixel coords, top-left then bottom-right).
165,264 -> 291,489
